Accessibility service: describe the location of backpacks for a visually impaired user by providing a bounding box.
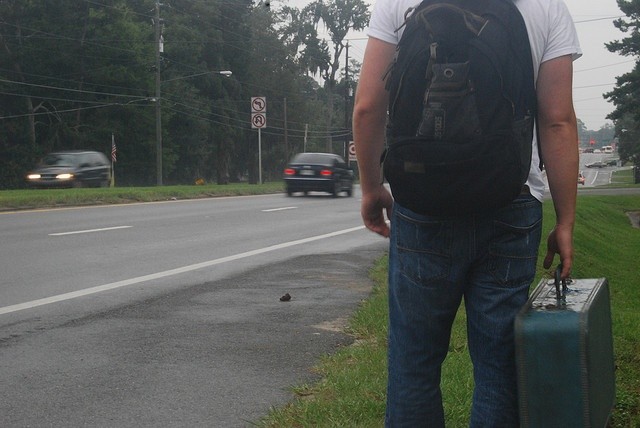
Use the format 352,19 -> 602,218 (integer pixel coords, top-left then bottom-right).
380,0 -> 542,218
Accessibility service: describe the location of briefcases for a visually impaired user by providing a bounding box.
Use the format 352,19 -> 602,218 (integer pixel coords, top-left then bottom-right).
514,264 -> 616,428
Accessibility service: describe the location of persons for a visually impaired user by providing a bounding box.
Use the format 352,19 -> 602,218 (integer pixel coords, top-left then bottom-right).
352,1 -> 583,428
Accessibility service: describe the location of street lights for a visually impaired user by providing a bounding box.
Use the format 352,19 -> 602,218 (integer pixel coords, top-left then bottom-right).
155,70 -> 232,186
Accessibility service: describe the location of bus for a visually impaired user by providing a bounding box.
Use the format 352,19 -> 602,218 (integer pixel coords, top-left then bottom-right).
602,146 -> 614,153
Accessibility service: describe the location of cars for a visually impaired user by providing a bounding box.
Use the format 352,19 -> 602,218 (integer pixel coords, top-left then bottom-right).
583,148 -> 594,153
282,152 -> 354,197
577,170 -> 586,185
26,151 -> 112,188
593,149 -> 601,153
585,161 -> 607,168
607,160 -> 617,166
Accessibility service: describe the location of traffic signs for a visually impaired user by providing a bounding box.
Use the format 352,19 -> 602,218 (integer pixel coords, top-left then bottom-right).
251,113 -> 267,129
250,97 -> 266,112
348,141 -> 357,161
